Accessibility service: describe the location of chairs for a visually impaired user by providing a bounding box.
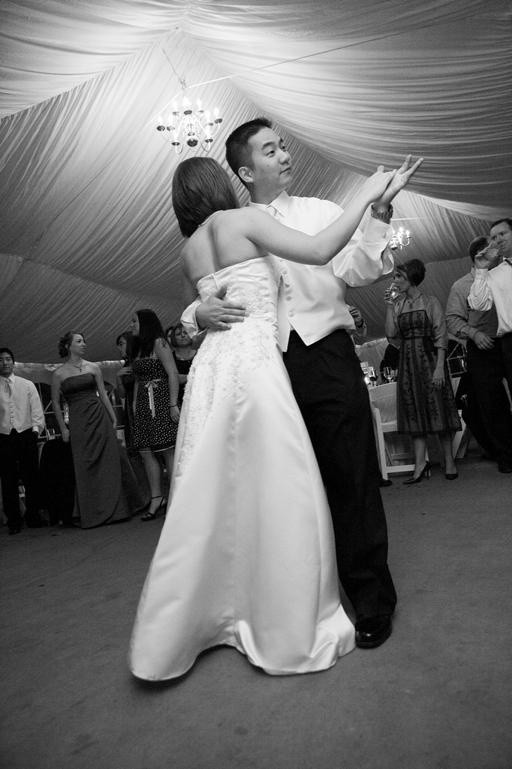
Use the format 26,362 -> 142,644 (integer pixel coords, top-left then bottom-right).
448,376 -> 474,462
367,380 -> 435,483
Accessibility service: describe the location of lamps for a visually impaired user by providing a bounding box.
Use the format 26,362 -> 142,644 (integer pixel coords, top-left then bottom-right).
388,223 -> 413,254
151,85 -> 227,159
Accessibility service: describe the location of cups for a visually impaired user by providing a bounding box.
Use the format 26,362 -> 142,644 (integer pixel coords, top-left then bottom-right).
46,429 -> 55,441
485,240 -> 499,260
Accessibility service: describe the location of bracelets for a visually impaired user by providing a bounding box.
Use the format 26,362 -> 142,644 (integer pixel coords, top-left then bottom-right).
370,202 -> 394,220
357,318 -> 364,330
168,403 -> 178,408
118,384 -> 124,386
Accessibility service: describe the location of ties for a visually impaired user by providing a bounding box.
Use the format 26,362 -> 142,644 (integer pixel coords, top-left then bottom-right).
266,206 -> 277,218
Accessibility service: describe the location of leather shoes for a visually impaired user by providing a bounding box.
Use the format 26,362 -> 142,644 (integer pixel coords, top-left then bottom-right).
355,614 -> 393,650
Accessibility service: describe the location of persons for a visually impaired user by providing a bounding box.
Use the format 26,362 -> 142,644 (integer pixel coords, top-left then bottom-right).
50,331 -> 150,531
172,322 -> 199,408
1,349 -> 55,537
128,156 -> 399,686
382,259 -> 463,485
125,307 -> 181,523
466,217 -> 512,474
344,303 -> 394,489
181,116 -> 428,650
165,326 -> 175,351
444,236 -> 503,461
115,331 -> 141,448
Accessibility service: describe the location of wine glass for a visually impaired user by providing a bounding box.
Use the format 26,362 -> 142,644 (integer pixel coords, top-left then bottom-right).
360,362 -> 395,386
385,283 -> 400,304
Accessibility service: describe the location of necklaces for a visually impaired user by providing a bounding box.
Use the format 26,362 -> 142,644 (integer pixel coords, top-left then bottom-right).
406,291 -> 422,309
69,360 -> 84,373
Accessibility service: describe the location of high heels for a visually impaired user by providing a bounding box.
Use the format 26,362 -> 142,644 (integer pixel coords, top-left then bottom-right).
402,460 -> 431,485
141,495 -> 167,521
445,471 -> 459,480
